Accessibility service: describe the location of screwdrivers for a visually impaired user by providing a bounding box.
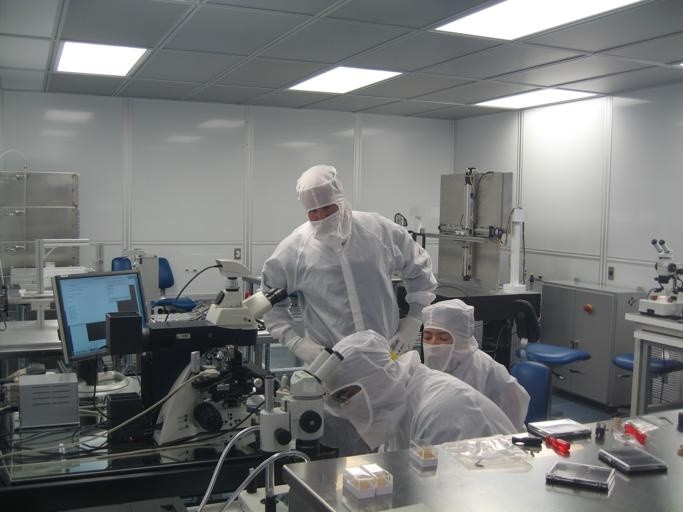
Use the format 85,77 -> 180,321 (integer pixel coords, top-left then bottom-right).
507,427 -> 570,449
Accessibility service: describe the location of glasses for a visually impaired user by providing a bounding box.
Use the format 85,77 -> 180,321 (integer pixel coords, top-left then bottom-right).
332,388 -> 352,407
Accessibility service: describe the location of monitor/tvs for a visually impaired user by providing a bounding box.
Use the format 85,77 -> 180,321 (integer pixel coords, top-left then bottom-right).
51,269 -> 149,393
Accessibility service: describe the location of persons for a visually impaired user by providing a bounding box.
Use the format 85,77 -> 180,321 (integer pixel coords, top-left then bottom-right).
317,329 -> 518,456
418,298 -> 533,435
255,161 -> 440,462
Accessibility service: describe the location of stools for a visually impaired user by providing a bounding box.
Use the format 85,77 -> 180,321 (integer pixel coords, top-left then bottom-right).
614,353 -> 683,416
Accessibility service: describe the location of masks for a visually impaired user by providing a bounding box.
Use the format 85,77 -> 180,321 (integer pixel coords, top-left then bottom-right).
339,391 -> 369,420
422,343 -> 453,370
309,212 -> 340,235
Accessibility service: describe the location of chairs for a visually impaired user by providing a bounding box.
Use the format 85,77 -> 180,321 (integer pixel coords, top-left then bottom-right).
506,298 -> 590,430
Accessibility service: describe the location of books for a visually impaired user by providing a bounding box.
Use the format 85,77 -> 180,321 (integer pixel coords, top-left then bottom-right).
544,460 -> 615,492
526,416 -> 593,441
597,448 -> 667,476
